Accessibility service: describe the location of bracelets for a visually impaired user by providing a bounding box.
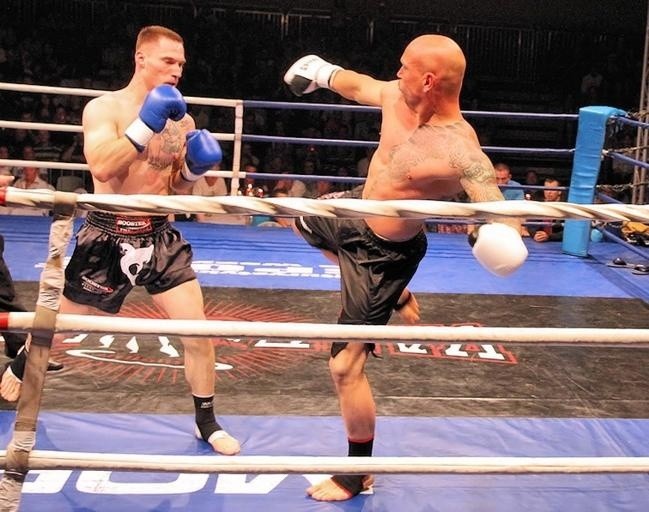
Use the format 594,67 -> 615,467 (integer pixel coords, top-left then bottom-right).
330,69 -> 344,88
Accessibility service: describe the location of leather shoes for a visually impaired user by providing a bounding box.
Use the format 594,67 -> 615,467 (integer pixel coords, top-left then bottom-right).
47,359 -> 64,371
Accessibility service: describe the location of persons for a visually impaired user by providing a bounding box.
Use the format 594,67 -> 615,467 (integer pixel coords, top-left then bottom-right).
282,34 -> 528,503
1,25 -> 241,455
179,55 -> 381,226
1,52 -> 131,208
429,165 -> 621,244
0,234 -> 64,371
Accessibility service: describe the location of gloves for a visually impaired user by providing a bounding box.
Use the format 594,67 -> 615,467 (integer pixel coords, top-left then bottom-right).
125,84 -> 187,155
468,222 -> 529,281
283,54 -> 345,97
179,128 -> 223,184
